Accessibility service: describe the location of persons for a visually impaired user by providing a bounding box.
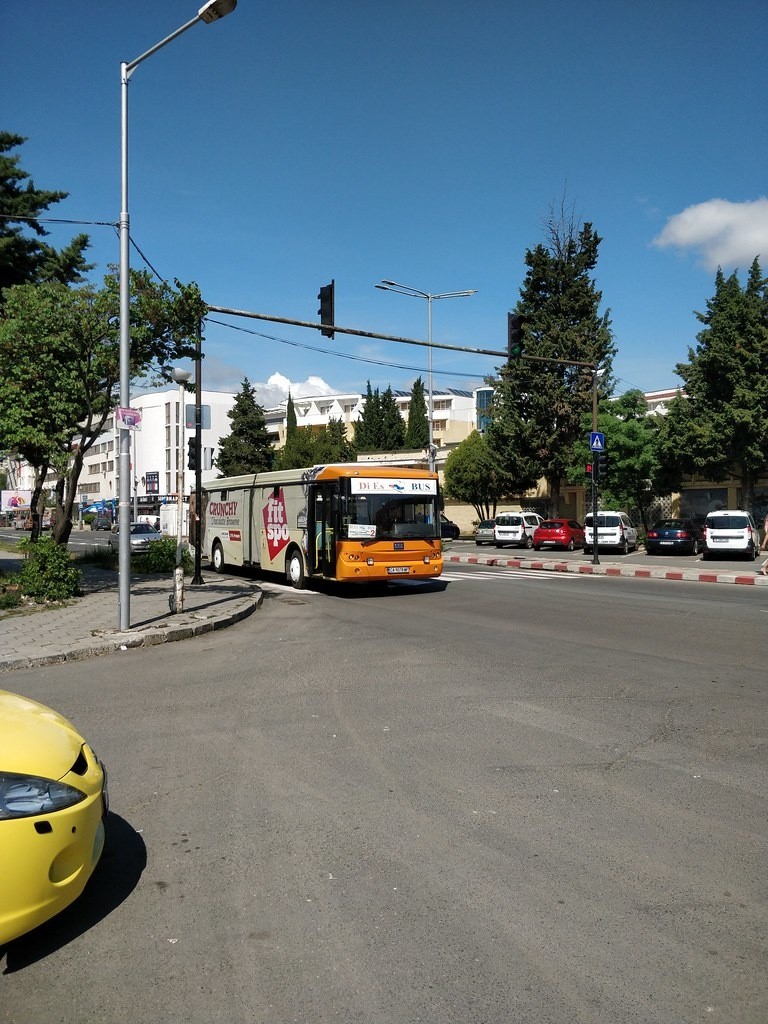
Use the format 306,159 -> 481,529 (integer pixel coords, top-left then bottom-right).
145,518 -> 153,526
761,515 -> 768,576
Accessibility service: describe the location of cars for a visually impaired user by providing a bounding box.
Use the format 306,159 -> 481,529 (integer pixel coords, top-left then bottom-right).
475,519 -> 497,545
108,522 -> 164,556
90,518 -> 112,532
440,513 -> 461,540
534,518 -> 586,552
1,688 -> 109,953
645,519 -> 704,556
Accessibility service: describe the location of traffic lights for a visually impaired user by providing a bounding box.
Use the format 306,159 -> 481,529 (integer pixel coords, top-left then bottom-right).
507,312 -> 526,358
586,463 -> 593,474
318,283 -> 334,337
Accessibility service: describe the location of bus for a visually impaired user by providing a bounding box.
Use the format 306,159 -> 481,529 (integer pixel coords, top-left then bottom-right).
187,463 -> 444,590
10,506 -> 53,531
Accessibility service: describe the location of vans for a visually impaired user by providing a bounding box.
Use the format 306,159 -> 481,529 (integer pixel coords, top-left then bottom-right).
703,509 -> 761,561
584,510 -> 640,555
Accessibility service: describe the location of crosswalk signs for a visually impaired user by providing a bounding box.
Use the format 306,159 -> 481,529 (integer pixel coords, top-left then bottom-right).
590,431 -> 605,452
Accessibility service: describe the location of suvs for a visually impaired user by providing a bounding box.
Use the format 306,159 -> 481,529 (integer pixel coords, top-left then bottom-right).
493,511 -> 545,549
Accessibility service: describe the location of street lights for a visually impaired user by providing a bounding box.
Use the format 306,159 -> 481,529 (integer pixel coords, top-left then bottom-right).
116,0 -> 238,630
170,366 -> 193,614
375,280 -> 481,538
